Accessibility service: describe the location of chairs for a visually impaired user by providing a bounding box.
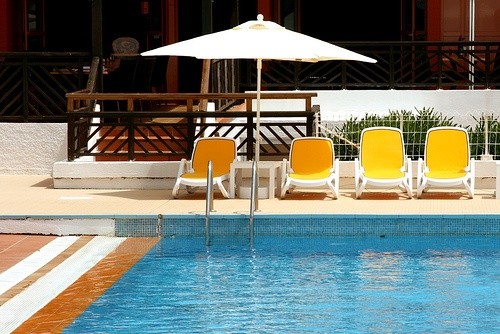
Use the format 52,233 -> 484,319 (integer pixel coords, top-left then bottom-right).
355,126 -> 413,199
172,137 -> 238,198
280,137 -> 340,198
417,126 -> 475,199
25,44 -> 157,125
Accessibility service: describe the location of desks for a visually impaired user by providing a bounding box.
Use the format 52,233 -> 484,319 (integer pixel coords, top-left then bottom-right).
230,161 -> 285,199
49,66 -> 112,116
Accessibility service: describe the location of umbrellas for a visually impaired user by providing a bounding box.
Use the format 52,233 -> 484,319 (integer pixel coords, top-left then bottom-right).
141,14 -> 378,211
